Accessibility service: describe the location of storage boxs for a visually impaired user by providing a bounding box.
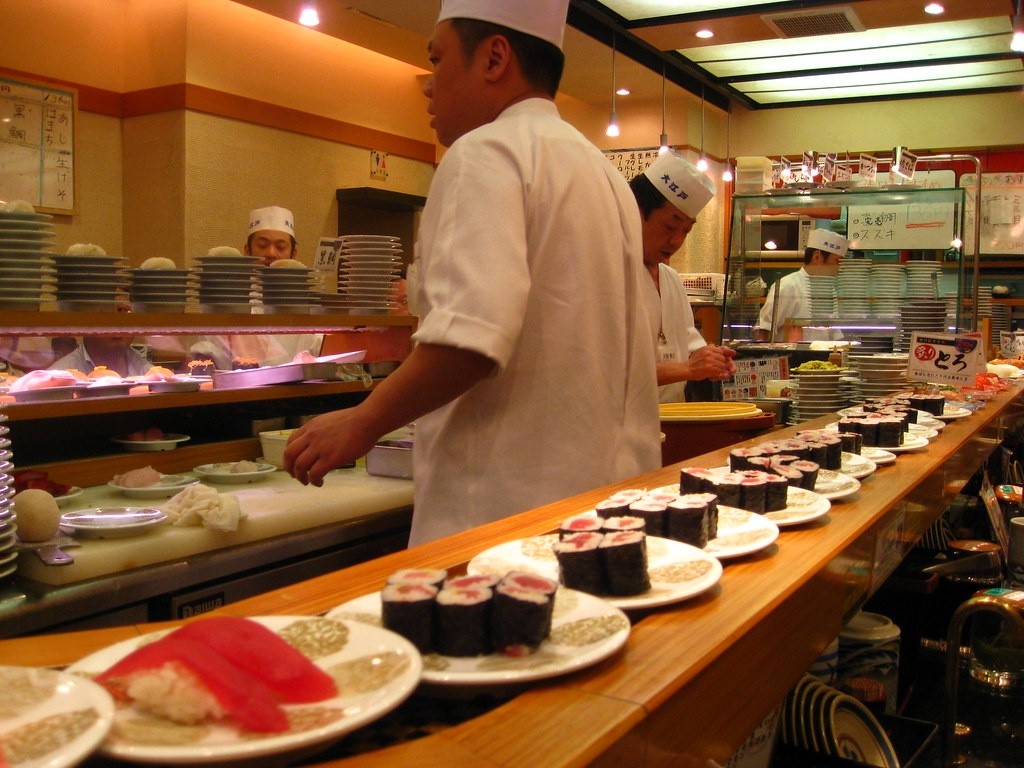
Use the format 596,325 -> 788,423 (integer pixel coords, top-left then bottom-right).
735,157 -> 773,171
717,186 -> 977,357
255,427 -> 309,476
365,442 -> 418,480
735,170 -> 774,185
735,184 -> 773,195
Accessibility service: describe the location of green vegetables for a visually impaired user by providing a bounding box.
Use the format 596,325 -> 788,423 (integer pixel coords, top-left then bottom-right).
795,360 -> 850,371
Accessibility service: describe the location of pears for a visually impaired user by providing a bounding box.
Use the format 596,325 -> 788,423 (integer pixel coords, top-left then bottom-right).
13,489 -> 59,541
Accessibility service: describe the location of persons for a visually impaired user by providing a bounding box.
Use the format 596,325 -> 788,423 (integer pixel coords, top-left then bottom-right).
190,205 -> 324,371
282,0 -> 665,553
620,150 -> 739,405
753,228 -> 851,343
0,283 -> 167,381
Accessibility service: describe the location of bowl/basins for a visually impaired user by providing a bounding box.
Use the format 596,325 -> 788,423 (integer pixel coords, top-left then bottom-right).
991,292 -> 1011,298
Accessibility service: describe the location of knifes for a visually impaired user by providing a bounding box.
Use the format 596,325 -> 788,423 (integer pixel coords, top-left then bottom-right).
15,535 -> 81,566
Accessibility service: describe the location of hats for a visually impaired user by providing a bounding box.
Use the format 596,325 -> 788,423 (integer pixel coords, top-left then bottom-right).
247,206 -> 294,237
435,0 -> 569,51
643,149 -> 717,218
806,228 -> 850,257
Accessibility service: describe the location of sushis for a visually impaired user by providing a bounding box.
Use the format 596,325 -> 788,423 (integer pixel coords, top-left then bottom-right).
381,566 -> 557,657
551,467 -> 787,597
729,391 -> 944,489
66,355 -> 261,384
94,617 -> 339,732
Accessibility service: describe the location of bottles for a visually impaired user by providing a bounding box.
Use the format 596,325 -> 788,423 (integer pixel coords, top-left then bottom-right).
828,345 -> 841,365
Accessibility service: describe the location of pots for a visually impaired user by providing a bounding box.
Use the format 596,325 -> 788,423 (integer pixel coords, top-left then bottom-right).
736,398 -> 792,424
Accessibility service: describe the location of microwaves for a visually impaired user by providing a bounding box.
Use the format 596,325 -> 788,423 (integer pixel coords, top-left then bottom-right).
744,215 -> 816,259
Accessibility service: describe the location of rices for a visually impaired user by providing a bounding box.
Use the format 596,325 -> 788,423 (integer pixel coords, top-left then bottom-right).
141,256 -> 176,269
65,242 -> 107,257
269,258 -> 308,267
208,246 -> 242,257
0,199 -> 36,212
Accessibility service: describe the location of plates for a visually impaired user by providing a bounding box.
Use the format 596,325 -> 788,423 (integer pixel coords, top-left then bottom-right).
323,588 -> 630,683
578,504 -> 780,558
765,181 -> 921,196
468,532 -> 721,609
0,615 -> 422,768
0,369 -> 233,400
650,484 -> 832,525
0,413 -> 278,583
777,671 -> 901,768
707,467 -> 858,499
786,259 -> 1024,478
0,212 -> 404,315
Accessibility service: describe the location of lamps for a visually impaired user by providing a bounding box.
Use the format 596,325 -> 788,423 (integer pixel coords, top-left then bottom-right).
721,114 -> 733,183
1010,0 -> 1024,52
607,47 -> 621,139
696,90 -> 710,172
657,67 -> 670,156
298,0 -> 320,27
